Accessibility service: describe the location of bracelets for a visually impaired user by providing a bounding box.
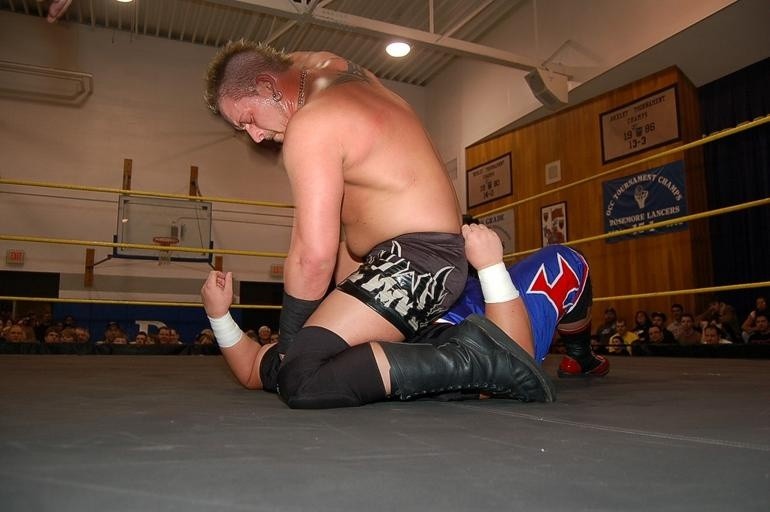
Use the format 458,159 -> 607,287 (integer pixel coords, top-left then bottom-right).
277,291 -> 323,354
476,261 -> 520,304
205,310 -> 241,348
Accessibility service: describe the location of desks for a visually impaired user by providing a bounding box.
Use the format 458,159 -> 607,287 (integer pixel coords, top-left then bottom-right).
0,336 -> 222,359
625,339 -> 769,360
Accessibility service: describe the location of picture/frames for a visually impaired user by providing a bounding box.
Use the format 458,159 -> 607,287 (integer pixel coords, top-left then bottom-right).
540,201 -> 568,250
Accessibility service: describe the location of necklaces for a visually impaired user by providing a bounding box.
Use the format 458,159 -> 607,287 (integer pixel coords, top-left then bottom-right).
299,70 -> 306,109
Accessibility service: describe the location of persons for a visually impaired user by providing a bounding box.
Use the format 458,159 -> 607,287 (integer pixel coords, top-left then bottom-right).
590,298 -> 769,357
554,338 -> 567,355
200,223 -> 610,390
202,37 -> 555,410
243,325 -> 280,346
1,311 -> 217,345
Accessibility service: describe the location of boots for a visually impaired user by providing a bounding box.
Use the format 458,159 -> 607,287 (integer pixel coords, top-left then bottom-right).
560,319 -> 615,376
372,313 -> 559,411
